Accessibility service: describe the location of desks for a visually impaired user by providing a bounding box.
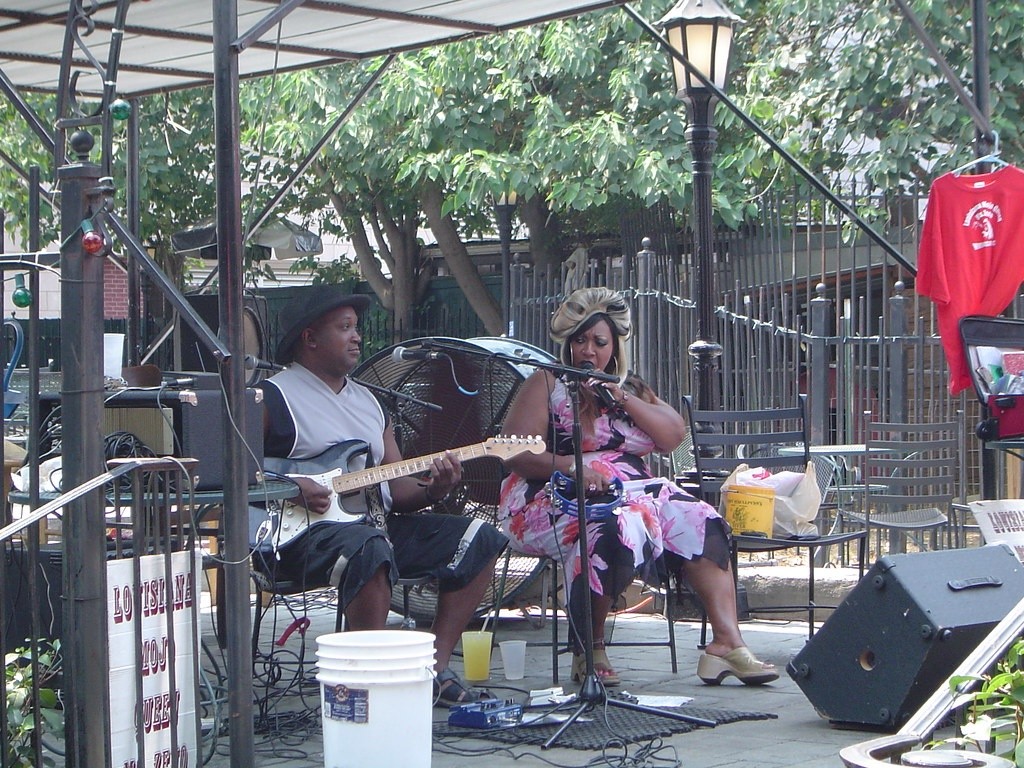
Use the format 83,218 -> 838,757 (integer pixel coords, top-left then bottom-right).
777,443 -> 896,567
9,478 -> 299,650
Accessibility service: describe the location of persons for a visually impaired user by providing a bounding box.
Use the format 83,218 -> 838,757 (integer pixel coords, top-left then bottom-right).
498,287 -> 780,684
251,287 -> 509,710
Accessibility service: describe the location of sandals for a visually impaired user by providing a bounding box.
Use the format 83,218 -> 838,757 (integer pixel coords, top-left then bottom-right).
433,668 -> 500,710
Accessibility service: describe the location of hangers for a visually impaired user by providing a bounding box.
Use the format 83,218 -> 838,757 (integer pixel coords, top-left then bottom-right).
953,130 -> 1008,173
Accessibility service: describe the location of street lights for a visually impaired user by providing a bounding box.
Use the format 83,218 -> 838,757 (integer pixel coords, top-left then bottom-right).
651,0 -> 749,622
492,188 -> 516,334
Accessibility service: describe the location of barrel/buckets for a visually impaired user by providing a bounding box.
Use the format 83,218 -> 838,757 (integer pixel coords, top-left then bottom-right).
315,630 -> 438,768
723,485 -> 774,538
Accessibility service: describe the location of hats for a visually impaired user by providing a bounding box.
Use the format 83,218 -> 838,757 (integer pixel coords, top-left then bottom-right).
275,284 -> 372,355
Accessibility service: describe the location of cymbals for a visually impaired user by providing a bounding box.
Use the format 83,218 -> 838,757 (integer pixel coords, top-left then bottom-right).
543,470 -> 630,521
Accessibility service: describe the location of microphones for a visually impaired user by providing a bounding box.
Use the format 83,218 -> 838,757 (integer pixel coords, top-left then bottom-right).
244,355 -> 287,372
581,362 -> 616,406
391,346 -> 448,363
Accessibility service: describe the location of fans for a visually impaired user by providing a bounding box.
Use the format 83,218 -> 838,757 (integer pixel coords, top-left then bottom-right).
349,337 -> 569,625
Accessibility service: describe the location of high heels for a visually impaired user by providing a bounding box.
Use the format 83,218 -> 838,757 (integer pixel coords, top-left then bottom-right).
699,647 -> 780,691
572,642 -> 621,690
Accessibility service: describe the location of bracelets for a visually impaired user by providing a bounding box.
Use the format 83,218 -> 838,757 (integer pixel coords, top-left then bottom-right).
417,483 -> 439,503
569,462 -> 576,475
618,389 -> 629,409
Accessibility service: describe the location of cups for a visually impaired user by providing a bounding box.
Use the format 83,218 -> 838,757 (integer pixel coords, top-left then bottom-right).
461,630 -> 493,681
499,640 -> 527,679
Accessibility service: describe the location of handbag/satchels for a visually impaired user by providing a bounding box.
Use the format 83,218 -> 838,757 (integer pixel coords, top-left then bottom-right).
719,461 -> 823,540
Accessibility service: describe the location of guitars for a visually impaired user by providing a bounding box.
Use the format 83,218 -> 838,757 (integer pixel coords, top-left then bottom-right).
248,434 -> 546,553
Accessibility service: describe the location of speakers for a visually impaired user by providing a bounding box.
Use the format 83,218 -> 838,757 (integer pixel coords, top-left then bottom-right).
786,543 -> 1024,735
178,294 -> 268,386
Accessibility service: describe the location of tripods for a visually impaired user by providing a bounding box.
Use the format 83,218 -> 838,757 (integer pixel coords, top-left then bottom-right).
423,340 -> 718,751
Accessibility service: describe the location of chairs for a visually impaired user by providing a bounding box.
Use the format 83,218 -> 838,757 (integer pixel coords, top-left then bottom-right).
838,410 -> 989,567
250,564 -> 434,676
490,542 -> 678,682
673,393 -> 869,650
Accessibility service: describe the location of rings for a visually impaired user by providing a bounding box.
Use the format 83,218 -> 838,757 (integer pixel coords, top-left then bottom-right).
608,482 -> 616,491
589,483 -> 595,492
